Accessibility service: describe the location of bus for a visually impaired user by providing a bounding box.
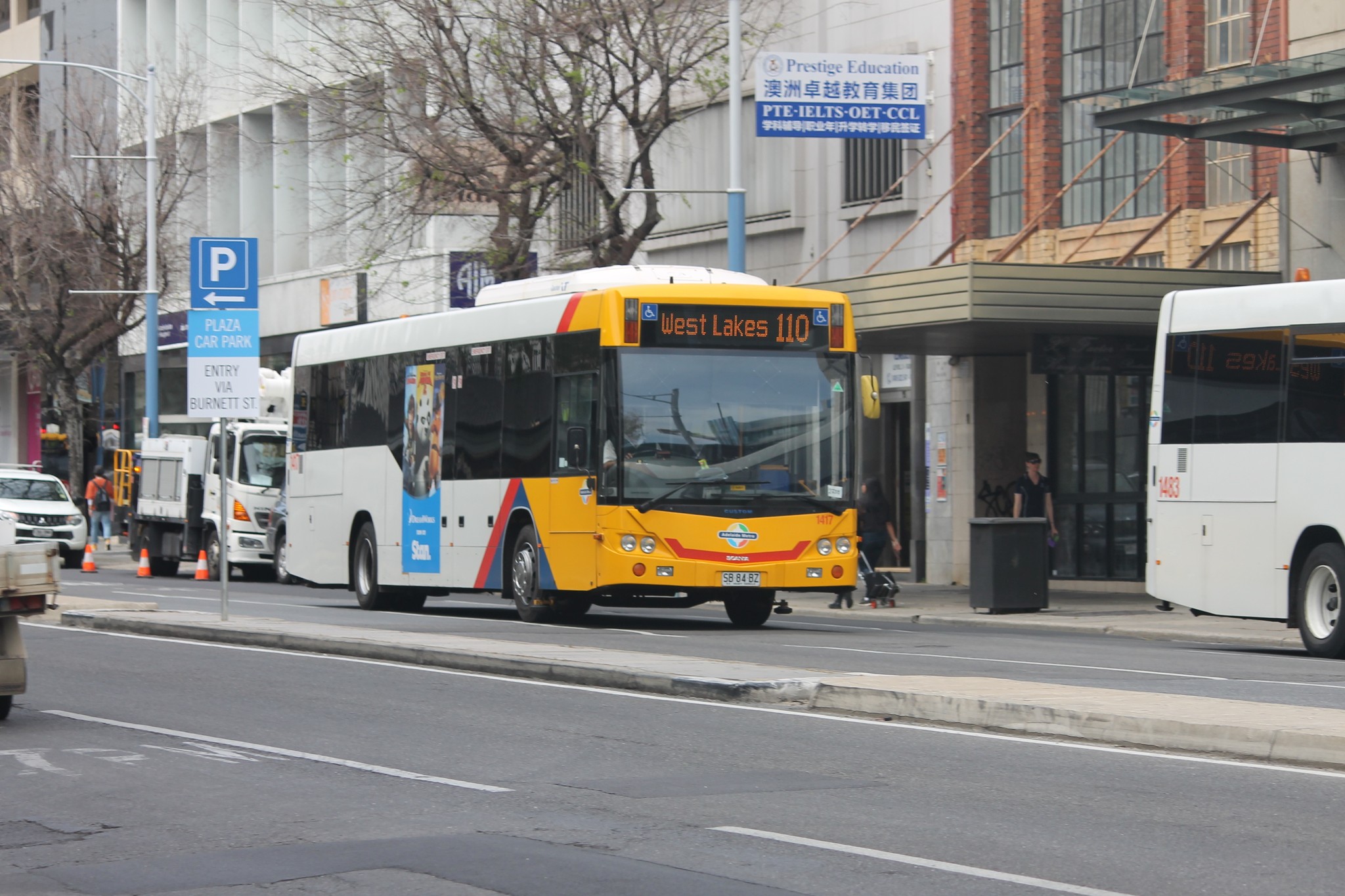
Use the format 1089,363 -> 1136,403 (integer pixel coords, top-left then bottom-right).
283,265 -> 883,628
1150,278 -> 1344,660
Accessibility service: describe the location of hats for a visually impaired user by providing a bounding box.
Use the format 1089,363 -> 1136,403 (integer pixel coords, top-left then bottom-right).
1025,452 -> 1041,462
93,464 -> 105,474
861,477 -> 880,487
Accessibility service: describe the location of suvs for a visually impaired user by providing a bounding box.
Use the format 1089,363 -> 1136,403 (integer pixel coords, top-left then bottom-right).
0,467 -> 87,568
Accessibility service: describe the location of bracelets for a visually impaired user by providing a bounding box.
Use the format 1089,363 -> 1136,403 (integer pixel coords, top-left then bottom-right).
1049,522 -> 1055,524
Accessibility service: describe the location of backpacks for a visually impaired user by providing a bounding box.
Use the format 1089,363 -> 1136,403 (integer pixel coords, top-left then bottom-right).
90,479 -> 112,511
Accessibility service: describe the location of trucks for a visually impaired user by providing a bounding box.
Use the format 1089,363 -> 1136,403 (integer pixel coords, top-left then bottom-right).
113,420 -> 286,580
0,529 -> 60,720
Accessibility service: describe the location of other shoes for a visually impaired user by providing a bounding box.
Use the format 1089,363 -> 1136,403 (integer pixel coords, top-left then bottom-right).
857,596 -> 871,606
104,539 -> 111,551
91,544 -> 98,551
846,598 -> 853,608
828,602 -> 842,609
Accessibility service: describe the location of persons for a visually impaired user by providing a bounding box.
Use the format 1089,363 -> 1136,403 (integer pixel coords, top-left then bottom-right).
602,412 -> 665,469
1013,453 -> 1058,537
85,465 -> 115,551
828,478 -> 902,609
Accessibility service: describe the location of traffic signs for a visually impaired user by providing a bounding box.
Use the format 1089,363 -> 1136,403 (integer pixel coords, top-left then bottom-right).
190,237 -> 258,309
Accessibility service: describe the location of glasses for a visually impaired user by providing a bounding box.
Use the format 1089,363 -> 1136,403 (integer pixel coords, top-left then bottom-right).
1029,460 -> 1041,464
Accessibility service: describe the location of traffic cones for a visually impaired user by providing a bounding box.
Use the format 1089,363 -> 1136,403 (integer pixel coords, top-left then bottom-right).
79,544 -> 98,573
190,550 -> 211,581
134,549 -> 154,578
